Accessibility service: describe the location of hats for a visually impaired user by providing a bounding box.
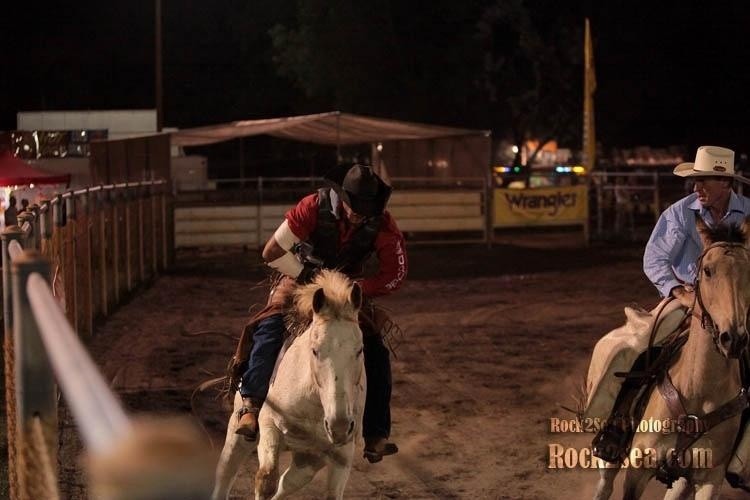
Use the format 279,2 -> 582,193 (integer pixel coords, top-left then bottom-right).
672,145 -> 750,184
321,164 -> 392,231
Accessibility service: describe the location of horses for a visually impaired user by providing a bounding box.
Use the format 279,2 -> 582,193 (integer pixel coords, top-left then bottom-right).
567,205 -> 748,499
207,268 -> 376,500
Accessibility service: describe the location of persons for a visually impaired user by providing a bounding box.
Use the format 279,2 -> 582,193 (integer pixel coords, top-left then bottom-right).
5,196 -> 29,227
234,162 -> 408,456
591,145 -> 750,489
614,174 -> 641,233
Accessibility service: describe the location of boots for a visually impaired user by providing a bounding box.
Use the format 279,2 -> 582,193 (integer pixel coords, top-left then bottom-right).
592,346 -> 663,464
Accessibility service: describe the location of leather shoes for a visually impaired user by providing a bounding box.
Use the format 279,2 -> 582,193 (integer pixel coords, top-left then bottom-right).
364,442 -> 398,463
234,406 -> 259,442
